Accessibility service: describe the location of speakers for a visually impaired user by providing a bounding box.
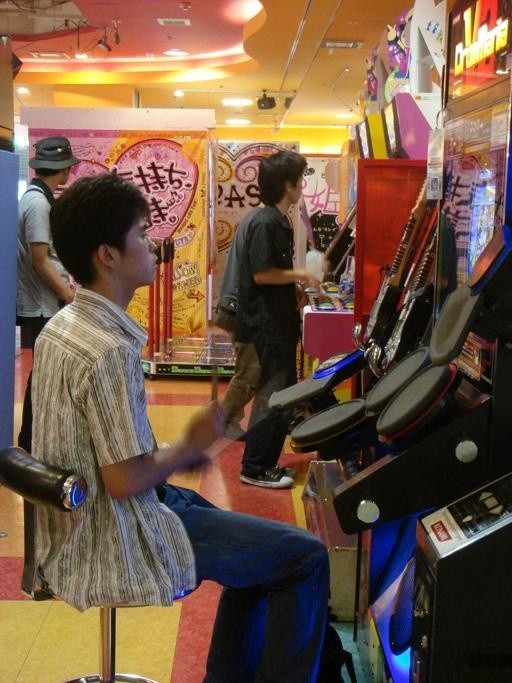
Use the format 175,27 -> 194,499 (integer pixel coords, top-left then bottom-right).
256,97 -> 276,109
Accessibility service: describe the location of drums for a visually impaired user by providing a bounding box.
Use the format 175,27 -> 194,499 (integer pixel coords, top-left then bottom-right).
376,363 -> 463,456
428,281 -> 490,365
290,398 -> 369,454
268,371 -> 337,412
364,346 -> 431,422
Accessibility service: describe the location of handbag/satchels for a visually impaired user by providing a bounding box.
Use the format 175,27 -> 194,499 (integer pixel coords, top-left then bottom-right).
316,625 -> 357,682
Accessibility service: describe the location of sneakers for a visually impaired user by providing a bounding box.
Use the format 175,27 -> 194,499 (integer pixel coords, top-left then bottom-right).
224,420 -> 246,440
275,464 -> 296,477
239,467 -> 295,487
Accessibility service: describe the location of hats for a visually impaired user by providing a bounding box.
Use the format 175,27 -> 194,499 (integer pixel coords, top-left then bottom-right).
28,136 -> 81,169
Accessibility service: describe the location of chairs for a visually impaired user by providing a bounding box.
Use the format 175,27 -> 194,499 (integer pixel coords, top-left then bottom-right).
0,446 -> 170,683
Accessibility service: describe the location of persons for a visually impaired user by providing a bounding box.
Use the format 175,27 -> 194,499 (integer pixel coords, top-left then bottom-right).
27,171 -> 333,682
15,134 -> 76,454
230,147 -> 324,488
210,201 -> 265,442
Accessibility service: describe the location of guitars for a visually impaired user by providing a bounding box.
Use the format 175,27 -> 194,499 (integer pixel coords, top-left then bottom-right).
362,175 -> 430,348
376,211 -> 439,370
